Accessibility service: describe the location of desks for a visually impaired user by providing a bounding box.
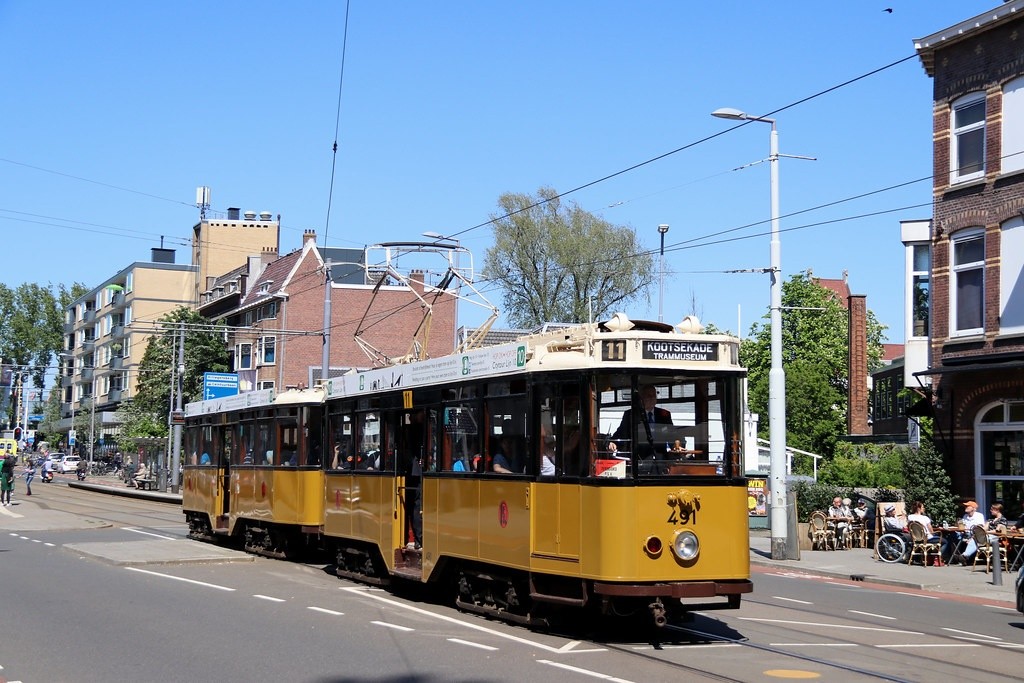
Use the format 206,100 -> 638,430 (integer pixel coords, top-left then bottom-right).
826,517 -> 853,550
1006,534 -> 1024,573
939,526 -> 969,567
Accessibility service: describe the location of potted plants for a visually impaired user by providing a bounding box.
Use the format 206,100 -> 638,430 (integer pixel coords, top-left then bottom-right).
913,286 -> 929,338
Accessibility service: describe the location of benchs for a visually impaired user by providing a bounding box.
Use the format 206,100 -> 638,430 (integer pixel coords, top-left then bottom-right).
135,478 -> 154,490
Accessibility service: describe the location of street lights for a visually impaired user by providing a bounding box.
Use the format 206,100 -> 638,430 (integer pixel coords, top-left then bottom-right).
658,224 -> 669,323
712,107 -> 826,560
58,353 -> 96,476
422,232 -> 459,351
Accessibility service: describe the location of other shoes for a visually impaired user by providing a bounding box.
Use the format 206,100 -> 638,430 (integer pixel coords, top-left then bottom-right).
958,555 -> 968,566
934,557 -> 944,566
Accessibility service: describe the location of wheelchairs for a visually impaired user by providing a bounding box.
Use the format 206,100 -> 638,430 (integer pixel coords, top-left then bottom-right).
875,514 -> 913,563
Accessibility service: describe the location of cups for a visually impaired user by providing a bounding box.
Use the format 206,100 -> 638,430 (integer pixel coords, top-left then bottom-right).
957,518 -> 963,529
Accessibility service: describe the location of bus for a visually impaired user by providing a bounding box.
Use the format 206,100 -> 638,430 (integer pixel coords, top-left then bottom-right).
181,242 -> 753,632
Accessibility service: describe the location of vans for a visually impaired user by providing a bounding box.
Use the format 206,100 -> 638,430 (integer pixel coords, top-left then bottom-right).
0,438 -> 19,463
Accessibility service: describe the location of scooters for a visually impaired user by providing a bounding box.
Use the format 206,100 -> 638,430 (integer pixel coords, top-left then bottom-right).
41,467 -> 53,483
75,465 -> 87,481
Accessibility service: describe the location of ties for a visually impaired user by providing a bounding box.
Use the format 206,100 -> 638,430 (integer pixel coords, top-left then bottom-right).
648,412 -> 655,435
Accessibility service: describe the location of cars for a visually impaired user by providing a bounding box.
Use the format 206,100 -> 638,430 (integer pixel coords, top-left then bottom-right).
49,453 -> 82,474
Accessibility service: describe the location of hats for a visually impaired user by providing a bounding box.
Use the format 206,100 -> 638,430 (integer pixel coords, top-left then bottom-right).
963,501 -> 978,509
857,498 -> 867,504
883,505 -> 897,513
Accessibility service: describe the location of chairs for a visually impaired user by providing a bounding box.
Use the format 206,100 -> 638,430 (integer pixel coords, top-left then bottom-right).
908,521 -> 942,568
970,526 -> 1008,573
810,510 -> 869,549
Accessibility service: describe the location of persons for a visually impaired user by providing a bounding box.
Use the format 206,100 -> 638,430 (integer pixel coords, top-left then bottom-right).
884,501 -> 1024,567
608,385 -> 687,476
451,422 -> 580,475
244,437 -> 317,465
0,452 -> 16,506
130,463 -> 146,489
191,441 -> 213,465
331,410 -> 436,549
76,458 -> 87,475
20,460 -> 36,495
41,458 -> 53,479
829,497 -> 868,549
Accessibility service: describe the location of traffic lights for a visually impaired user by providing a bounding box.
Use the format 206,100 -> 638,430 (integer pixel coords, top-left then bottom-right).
13,427 -> 22,442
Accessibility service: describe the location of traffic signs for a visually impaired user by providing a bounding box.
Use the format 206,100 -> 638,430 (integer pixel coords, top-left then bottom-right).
204,372 -> 239,400
